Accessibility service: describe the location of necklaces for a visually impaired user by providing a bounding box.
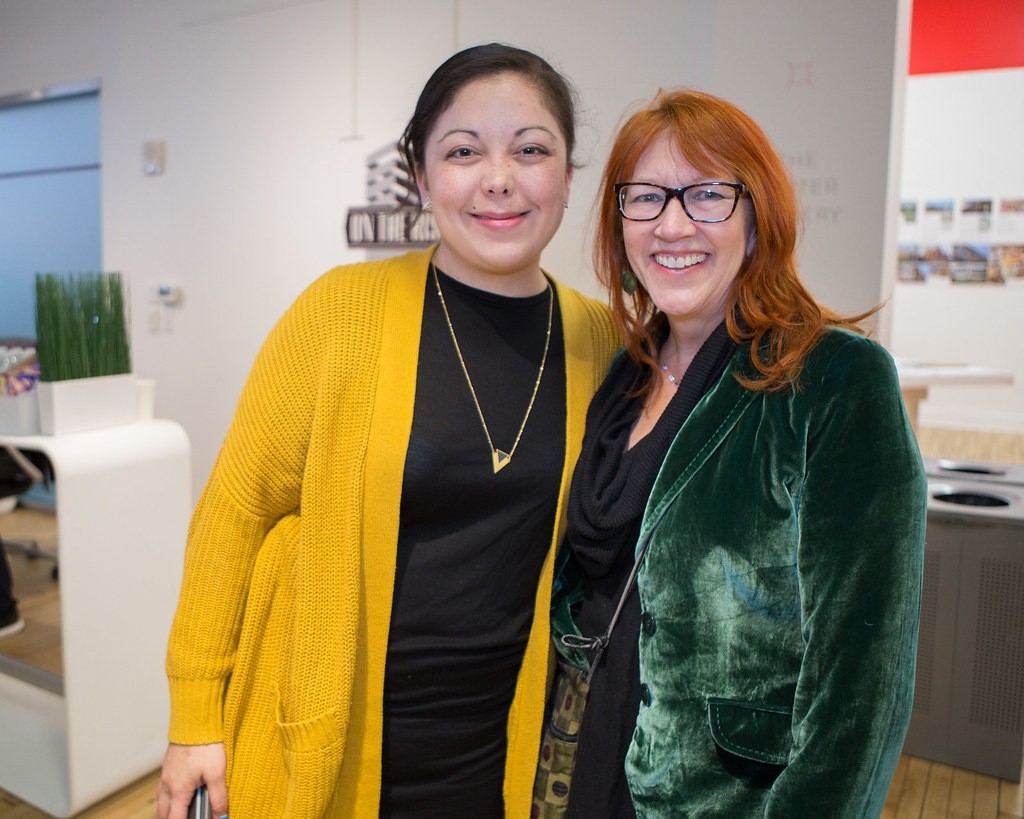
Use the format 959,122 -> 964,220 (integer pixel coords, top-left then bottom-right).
660,347 -> 679,386
430,256 -> 554,472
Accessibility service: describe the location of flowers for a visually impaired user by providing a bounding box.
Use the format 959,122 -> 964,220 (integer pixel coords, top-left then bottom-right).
0,346 -> 38,395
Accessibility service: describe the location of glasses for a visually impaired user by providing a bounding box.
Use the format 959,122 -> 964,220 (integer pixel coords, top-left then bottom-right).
615,181 -> 746,223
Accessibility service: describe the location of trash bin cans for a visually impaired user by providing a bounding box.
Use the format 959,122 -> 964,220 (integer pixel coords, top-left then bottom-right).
900,475 -> 1024,786
922,459 -> 1024,486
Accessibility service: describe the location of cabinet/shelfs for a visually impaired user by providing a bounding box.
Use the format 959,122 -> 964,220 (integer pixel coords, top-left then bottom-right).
0,425 -> 192,819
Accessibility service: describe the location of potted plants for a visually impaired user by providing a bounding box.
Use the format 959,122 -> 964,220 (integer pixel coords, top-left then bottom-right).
30,271 -> 142,433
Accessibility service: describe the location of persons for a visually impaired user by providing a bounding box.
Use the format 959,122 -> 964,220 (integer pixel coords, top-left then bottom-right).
159,41 -> 627,819
529,87 -> 929,819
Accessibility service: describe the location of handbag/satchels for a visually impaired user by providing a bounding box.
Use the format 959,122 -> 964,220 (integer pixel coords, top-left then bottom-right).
531,662 -> 592,819
